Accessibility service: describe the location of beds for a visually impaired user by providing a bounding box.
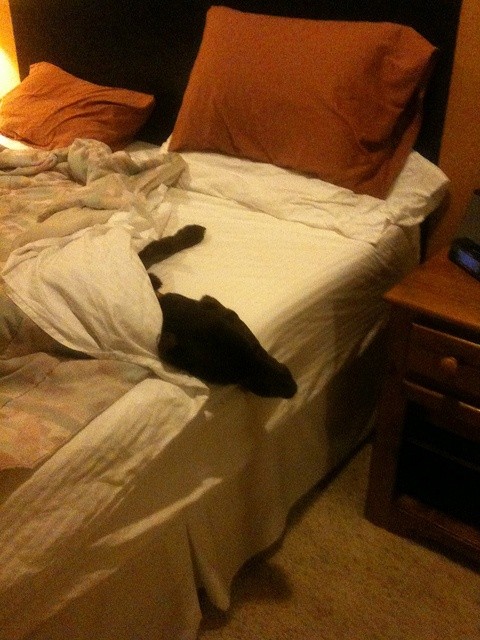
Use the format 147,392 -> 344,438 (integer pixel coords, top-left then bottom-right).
0,137 -> 450,640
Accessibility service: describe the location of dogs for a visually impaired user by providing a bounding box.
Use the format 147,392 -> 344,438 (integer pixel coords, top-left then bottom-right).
138,224 -> 299,400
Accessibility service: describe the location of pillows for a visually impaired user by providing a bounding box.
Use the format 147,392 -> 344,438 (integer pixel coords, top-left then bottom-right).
0,61 -> 155,151
168,5 -> 440,200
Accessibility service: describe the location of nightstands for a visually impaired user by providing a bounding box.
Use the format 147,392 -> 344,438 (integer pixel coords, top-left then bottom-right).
364,243 -> 478,572
